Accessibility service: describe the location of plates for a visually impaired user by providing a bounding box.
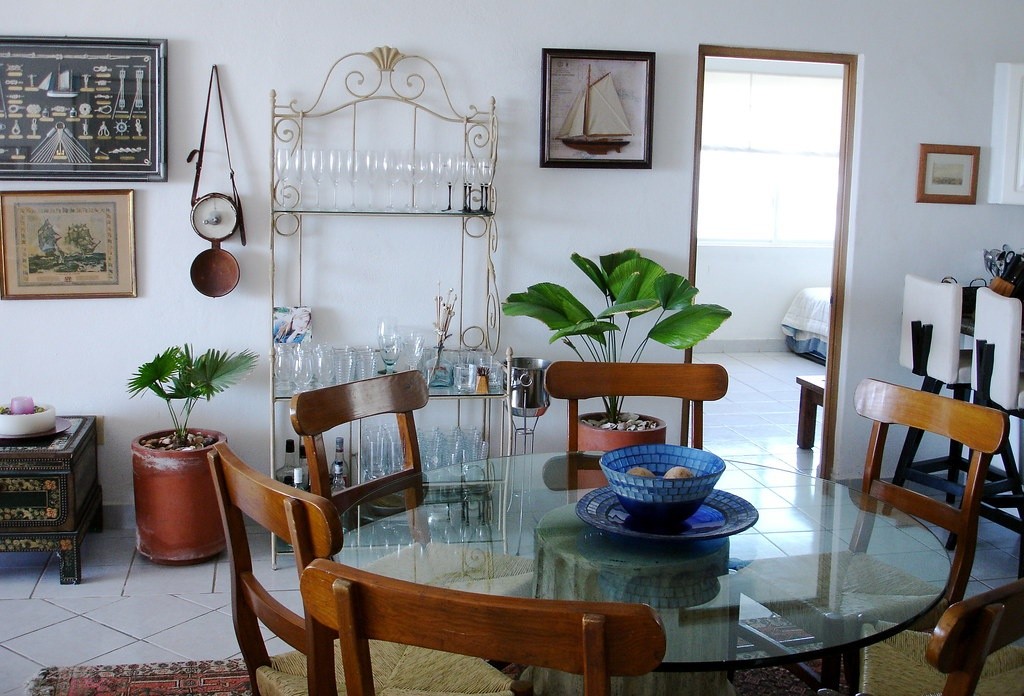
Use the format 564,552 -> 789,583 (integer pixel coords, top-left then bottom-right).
575,486 -> 758,538
0,417 -> 72,439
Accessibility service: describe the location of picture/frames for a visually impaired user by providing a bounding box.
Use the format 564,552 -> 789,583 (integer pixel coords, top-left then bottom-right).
540,48 -> 656,169
0,35 -> 169,182
0,190 -> 140,299
916,144 -> 981,206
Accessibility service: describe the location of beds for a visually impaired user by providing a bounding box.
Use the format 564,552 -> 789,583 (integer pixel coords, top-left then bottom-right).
781,286 -> 832,360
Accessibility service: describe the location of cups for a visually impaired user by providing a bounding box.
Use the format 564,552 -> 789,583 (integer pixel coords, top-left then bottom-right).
362,422 -> 490,482
273,321 -> 502,395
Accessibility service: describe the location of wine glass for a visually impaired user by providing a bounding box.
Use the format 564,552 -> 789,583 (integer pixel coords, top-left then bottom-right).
276,147 -> 494,215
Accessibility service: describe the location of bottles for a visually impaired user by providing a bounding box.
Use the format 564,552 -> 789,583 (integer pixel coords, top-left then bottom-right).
331,437 -> 350,488
275,439 -> 312,496
331,460 -> 347,536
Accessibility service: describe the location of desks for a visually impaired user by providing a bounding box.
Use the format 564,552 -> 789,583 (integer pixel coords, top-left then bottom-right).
307,453 -> 951,696
1,414 -> 103,585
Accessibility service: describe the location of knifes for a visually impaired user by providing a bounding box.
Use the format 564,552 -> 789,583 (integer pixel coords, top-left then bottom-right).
1000,252 -> 1024,286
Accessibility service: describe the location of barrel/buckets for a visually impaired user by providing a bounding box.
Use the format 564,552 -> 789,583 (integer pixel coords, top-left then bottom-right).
502,358 -> 551,408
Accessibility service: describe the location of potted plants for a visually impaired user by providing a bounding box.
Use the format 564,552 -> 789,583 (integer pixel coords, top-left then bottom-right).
501,248 -> 734,453
127,343 -> 259,566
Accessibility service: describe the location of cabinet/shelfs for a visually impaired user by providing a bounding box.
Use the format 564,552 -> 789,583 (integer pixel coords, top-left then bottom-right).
270,46 -> 500,571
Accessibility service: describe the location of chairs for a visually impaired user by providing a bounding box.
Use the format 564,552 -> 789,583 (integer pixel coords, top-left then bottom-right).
545,359 -> 728,491
959,284 -> 1024,524
290,369 -> 537,639
747,377 -> 1009,639
883,275 -> 1009,534
855,581 -> 1024,696
209,444 -> 525,696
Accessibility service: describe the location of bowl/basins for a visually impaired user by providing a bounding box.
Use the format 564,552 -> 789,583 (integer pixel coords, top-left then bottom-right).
599,444 -> 726,527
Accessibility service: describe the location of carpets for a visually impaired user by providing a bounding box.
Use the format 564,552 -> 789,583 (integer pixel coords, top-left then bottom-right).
28,618 -> 852,696
